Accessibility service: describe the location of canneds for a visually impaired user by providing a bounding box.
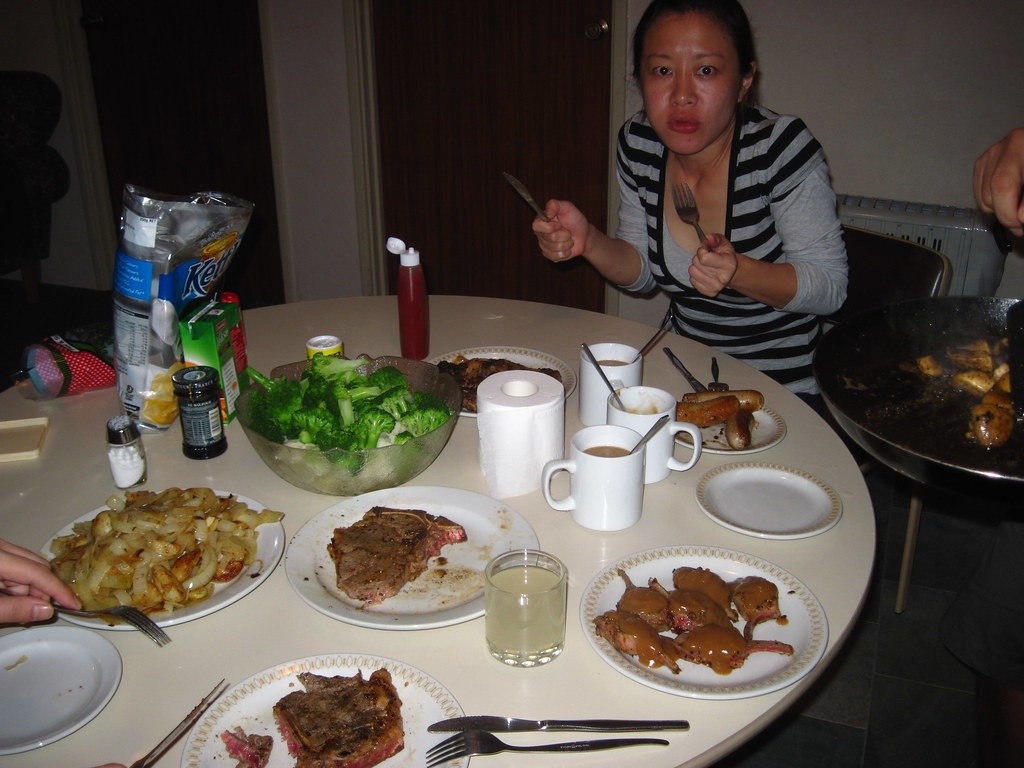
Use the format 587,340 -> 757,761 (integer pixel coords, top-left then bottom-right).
170,365 -> 228,460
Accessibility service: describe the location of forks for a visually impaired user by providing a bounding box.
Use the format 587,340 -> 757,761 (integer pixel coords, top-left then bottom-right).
709,356 -> 730,391
54,604 -> 171,647
670,183 -> 713,253
425,730 -> 669,766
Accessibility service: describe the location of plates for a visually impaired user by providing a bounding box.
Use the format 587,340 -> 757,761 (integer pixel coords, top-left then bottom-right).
1,624 -> 122,754
675,405 -> 787,456
38,487 -> 286,631
180,652 -> 471,768
578,545 -> 830,700
284,486 -> 540,630
429,346 -> 577,419
694,461 -> 842,540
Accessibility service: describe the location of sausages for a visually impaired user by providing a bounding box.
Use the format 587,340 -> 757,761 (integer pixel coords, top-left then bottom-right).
675,389 -> 765,451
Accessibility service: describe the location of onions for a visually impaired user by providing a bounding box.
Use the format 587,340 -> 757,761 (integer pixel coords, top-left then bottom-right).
48,486 -> 285,627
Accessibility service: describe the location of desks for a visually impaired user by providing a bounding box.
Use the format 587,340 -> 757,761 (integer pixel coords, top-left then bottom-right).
0,293 -> 876,768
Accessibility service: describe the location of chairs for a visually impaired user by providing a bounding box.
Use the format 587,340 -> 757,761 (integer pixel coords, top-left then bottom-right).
0,70 -> 71,303
821,224 -> 952,613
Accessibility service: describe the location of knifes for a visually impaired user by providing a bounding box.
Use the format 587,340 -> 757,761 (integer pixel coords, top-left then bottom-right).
128,678 -> 226,768
663,347 -> 708,392
428,716 -> 690,732
503,171 -> 548,221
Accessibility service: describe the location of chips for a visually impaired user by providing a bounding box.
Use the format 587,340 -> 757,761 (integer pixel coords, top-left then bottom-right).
143,361 -> 197,427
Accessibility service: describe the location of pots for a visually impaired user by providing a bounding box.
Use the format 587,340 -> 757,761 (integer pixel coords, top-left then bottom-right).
811,296 -> 1024,513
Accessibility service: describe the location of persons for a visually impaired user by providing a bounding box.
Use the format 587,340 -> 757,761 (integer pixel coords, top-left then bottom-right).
532,0 -> 851,459
0,538 -> 83,626
973,127 -> 1024,237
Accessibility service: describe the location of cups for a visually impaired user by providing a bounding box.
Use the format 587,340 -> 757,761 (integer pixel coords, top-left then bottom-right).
606,385 -> 701,484
579,342 -> 643,425
541,424 -> 645,531
484,549 -> 567,667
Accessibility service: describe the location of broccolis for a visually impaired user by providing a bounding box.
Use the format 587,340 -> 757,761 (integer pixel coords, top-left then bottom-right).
240,352 -> 452,475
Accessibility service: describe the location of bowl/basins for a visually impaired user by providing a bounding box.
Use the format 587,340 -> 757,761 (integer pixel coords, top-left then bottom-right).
233,355 -> 463,496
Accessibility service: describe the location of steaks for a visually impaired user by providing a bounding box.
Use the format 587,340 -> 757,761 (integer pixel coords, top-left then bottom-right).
435,356 -> 563,413
327,505 -> 467,602
220,669 -> 405,768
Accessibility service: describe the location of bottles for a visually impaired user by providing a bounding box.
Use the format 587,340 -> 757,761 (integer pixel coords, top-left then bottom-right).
104,415 -> 147,488
172,367 -> 229,460
387,237 -> 430,361
